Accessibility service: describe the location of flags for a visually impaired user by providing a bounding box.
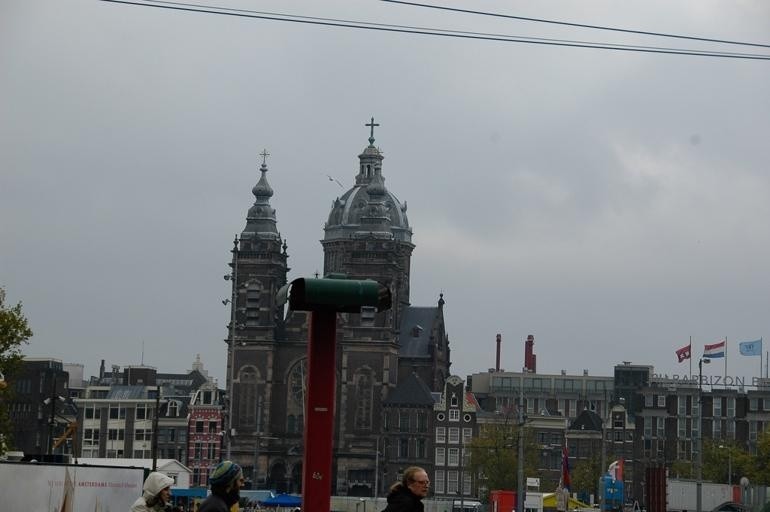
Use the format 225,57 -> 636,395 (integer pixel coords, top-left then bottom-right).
700,338 -> 727,360
559,441 -> 573,494
673,343 -> 692,363
737,337 -> 762,356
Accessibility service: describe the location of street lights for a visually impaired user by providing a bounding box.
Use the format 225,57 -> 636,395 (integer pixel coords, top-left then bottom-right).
717,445 -> 732,502
515,409 -> 531,512
41,393 -> 68,455
739,475 -> 751,508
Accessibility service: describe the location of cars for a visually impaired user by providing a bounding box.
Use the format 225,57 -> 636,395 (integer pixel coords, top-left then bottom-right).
708,501 -> 752,511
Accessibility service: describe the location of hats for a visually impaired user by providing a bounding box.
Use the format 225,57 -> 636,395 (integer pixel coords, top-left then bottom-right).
210,461 -> 242,488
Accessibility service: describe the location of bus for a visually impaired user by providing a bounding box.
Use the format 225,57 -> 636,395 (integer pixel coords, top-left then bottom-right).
453,500 -> 483,512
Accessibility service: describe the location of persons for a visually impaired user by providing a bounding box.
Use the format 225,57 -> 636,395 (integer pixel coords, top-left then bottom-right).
195,458 -> 246,511
126,471 -> 178,511
377,465 -> 431,512
